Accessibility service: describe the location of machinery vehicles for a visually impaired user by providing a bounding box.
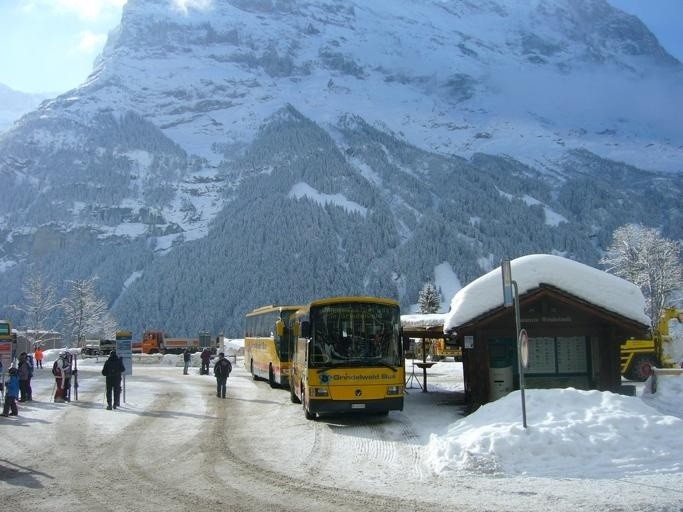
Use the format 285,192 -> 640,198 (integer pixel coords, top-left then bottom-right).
619,306 -> 683,384
400,333 -> 463,362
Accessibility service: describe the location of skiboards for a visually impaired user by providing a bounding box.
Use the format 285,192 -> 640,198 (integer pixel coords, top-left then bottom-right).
66,354 -> 74,401
73,353 -> 79,401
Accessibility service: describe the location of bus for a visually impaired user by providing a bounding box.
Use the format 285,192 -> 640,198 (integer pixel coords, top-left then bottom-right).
243,303 -> 305,389
287,296 -> 411,421
0,318 -> 18,393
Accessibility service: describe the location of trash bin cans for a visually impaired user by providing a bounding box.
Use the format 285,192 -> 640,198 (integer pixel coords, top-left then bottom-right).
489,357 -> 514,402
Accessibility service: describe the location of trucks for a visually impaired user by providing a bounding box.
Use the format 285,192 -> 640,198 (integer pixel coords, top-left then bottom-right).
141,329 -> 200,355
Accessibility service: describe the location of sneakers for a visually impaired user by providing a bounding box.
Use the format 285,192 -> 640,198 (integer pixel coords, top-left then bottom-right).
216,394 -> 225,398
108,404 -> 116,409
19,397 -> 32,402
0,413 -> 17,418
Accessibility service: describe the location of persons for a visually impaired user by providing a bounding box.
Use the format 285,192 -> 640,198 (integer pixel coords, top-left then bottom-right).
184,349 -> 190,375
102,350 -> 126,410
0,346 -> 77,417
200,348 -> 210,375
214,353 -> 232,398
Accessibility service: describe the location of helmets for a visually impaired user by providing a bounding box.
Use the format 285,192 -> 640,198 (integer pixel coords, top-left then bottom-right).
59,350 -> 73,359
9,367 -> 17,374
110,350 -> 116,355
